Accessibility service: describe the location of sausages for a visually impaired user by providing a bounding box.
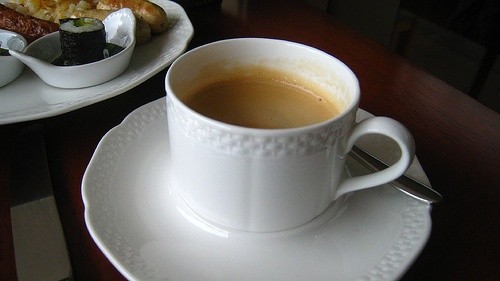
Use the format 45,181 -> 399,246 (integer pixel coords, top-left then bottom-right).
0,0 -> 168,45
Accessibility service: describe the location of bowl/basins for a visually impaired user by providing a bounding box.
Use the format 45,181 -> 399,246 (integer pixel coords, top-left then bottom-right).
8,8 -> 137,89
0,30 -> 28,88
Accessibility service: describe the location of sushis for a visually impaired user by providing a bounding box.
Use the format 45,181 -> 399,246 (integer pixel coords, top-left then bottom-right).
58,17 -> 109,65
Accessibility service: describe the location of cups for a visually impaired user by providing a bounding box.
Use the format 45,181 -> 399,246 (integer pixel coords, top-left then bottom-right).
164,38 -> 416,234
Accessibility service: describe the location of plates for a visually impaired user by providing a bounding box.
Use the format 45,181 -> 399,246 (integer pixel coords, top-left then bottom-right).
0,0 -> 195,124
80,95 -> 434,281
